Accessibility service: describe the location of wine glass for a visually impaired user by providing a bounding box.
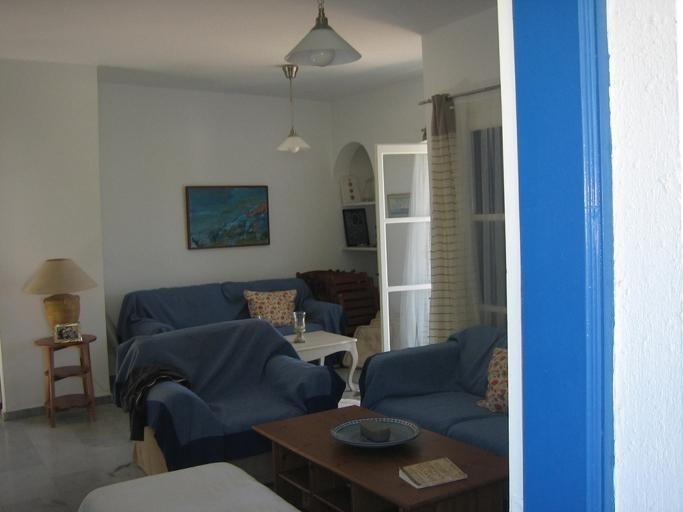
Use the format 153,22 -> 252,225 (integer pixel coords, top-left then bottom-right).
292,311 -> 306,343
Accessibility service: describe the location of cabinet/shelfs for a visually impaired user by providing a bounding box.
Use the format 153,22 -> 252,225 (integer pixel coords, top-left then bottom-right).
334,198 -> 378,253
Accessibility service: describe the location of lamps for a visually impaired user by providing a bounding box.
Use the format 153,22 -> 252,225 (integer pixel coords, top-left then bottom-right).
22,257 -> 100,337
283,1 -> 363,70
276,62 -> 312,156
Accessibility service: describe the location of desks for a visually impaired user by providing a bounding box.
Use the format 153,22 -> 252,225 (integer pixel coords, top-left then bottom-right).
32,334 -> 97,428
282,329 -> 360,393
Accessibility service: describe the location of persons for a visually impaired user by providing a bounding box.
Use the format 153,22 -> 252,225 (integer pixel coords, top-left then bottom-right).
59,329 -> 67,339
65,326 -> 77,338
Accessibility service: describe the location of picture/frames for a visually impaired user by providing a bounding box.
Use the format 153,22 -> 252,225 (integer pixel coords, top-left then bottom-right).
388,193 -> 412,217
181,183 -> 274,251
342,209 -> 369,248
52,322 -> 83,344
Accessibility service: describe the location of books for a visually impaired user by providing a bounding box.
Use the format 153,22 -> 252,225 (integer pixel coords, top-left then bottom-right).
398,456 -> 467,489
339,173 -> 363,207
340,206 -> 372,249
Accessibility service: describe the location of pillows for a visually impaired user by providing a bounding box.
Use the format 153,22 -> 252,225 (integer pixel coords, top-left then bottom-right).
472,343 -> 509,416
242,287 -> 295,326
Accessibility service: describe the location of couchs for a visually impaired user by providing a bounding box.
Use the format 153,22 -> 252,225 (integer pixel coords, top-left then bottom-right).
359,324 -> 508,462
120,276 -> 341,366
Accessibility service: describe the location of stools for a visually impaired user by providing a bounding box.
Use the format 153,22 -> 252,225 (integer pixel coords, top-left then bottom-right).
77,460 -> 301,512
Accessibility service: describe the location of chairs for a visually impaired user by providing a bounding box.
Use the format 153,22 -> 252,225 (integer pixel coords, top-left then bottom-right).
109,326 -> 347,484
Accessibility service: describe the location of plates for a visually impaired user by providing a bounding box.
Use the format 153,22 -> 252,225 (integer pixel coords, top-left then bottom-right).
329,415 -> 421,450
